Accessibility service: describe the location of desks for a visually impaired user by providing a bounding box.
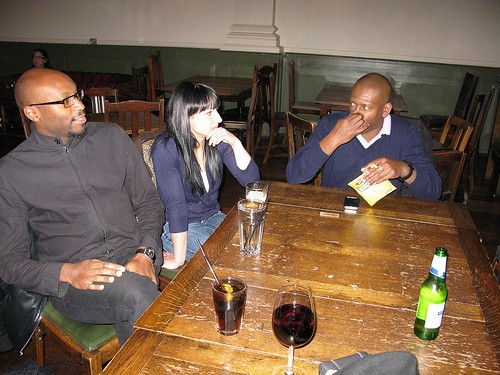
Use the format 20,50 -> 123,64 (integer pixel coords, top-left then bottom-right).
158,76 -> 252,144
94,327 -> 500,375
315,83 -> 409,120
133,178 -> 500,349
84,110 -> 165,137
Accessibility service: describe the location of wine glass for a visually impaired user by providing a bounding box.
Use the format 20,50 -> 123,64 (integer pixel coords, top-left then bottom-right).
272,285 -> 317,375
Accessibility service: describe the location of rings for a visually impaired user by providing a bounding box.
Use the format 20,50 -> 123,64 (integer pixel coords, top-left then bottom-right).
379,165 -> 383,172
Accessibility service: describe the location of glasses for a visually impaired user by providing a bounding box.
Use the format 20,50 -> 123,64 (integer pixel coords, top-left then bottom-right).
32,55 -> 43,58
28,87 -> 85,107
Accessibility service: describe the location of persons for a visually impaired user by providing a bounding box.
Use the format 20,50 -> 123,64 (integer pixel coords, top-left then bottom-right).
33,48 -> 53,68
148,81 -> 261,270
0,67 -> 166,347
286,72 -> 442,200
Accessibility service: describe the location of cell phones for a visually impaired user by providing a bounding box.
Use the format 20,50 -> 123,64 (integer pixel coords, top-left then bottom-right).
344,196 -> 359,209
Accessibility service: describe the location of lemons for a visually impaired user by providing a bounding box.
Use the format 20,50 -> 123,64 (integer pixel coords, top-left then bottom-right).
223,284 -> 233,301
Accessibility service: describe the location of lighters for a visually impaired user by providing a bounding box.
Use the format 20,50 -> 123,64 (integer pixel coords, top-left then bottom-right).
320,212 -> 339,217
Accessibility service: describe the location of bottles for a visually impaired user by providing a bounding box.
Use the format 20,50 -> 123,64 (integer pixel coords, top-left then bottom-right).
414,246 -> 448,341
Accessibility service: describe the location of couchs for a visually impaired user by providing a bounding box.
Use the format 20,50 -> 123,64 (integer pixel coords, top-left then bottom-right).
0,68 -> 157,134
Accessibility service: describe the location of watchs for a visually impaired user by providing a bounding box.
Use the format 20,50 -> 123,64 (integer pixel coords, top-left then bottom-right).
135,247 -> 156,265
398,160 -> 414,183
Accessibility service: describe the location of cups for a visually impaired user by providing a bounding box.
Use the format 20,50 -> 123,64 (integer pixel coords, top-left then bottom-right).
245,181 -> 269,203
238,199 -> 267,256
211,277 -> 247,336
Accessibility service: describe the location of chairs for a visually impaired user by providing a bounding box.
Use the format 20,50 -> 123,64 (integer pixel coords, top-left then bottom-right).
30,69 -> 500,375
